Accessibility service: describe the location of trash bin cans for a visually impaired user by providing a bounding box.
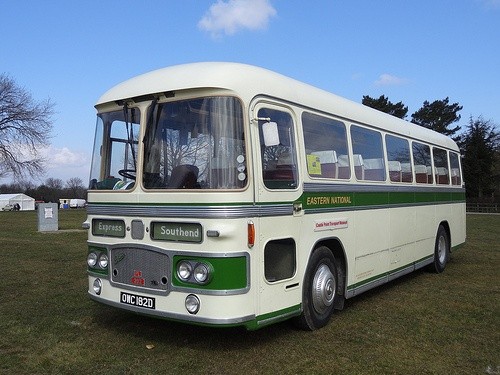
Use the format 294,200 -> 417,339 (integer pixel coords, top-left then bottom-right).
63,203 -> 68,209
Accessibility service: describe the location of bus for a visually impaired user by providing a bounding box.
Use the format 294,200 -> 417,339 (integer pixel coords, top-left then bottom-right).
83,59 -> 467,333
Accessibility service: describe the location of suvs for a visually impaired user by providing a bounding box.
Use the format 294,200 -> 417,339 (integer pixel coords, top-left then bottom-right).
3,203 -> 20,212
